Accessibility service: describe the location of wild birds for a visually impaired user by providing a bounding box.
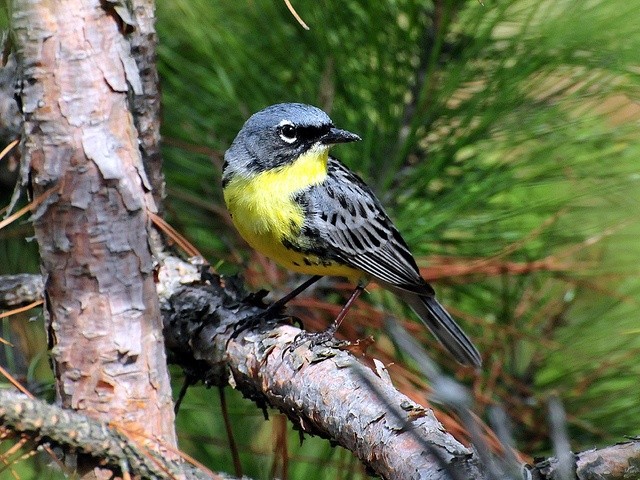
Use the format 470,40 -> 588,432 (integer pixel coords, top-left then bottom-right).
221,103 -> 482,368
